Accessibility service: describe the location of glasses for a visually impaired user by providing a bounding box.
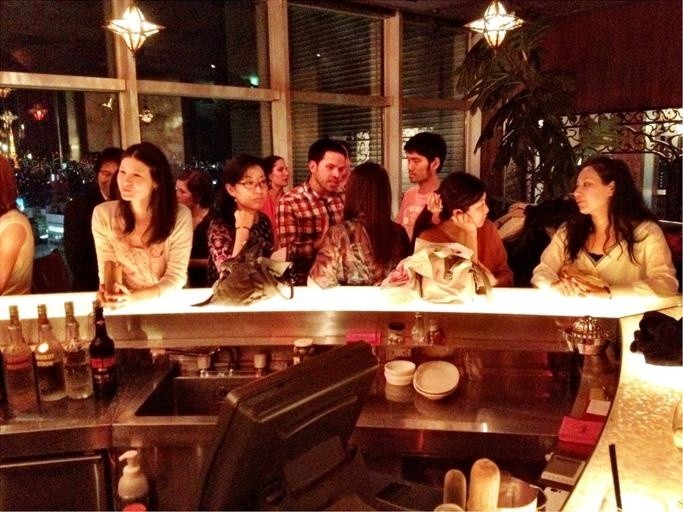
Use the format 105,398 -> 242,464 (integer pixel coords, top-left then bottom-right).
237,179 -> 272,189
100,170 -> 115,177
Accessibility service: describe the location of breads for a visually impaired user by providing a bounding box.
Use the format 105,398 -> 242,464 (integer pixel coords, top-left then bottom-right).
469,458 -> 499,510
435,505 -> 463,512
442,468 -> 467,509
499,470 -> 536,508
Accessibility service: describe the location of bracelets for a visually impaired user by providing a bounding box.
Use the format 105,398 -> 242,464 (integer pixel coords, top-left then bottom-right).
235,226 -> 252,230
604,286 -> 614,300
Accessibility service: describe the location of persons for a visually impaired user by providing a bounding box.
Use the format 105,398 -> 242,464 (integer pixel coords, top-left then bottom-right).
276,139 -> 351,284
64,147 -> 125,292
174,167 -> 215,286
261,155 -> 290,246
91,142 -> 194,310
530,156 -> 679,298
630,311 -> 683,368
396,132 -> 449,241
307,162 -> 411,287
206,156 -> 275,280
411,170 -> 514,287
0,154 -> 34,295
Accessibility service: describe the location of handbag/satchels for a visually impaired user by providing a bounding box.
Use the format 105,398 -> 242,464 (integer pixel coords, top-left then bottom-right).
381,246 -> 448,303
207,256 -> 293,306
424,244 -> 498,303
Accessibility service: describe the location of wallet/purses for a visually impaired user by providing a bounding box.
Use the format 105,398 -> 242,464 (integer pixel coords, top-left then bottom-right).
567,266 -> 613,302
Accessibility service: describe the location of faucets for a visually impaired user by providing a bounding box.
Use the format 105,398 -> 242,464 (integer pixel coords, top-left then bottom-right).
207,346 -> 240,377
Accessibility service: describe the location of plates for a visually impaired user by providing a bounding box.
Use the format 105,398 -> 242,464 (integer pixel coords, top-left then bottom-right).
413,360 -> 460,401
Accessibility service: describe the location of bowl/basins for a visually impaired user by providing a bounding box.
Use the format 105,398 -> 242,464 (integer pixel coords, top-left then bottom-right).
384,361 -> 415,385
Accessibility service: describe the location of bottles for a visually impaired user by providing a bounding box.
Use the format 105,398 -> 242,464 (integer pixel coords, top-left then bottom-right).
88,300 -> 102,340
428,319 -> 444,344
388,321 -> 406,346
4,320 -> 38,409
90,309 -> 115,401
64,300 -> 74,325
35,317 -> 65,402
37,304 -> 48,343
8,306 -> 25,346
293,338 -> 313,365
61,315 -> 92,400
411,312 -> 426,343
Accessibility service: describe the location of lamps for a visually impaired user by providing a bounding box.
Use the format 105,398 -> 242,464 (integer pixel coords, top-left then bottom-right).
102,6 -> 165,55
465,0 -> 523,57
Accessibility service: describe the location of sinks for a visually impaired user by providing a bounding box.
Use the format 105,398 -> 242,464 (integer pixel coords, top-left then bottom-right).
133,368 -> 260,418
